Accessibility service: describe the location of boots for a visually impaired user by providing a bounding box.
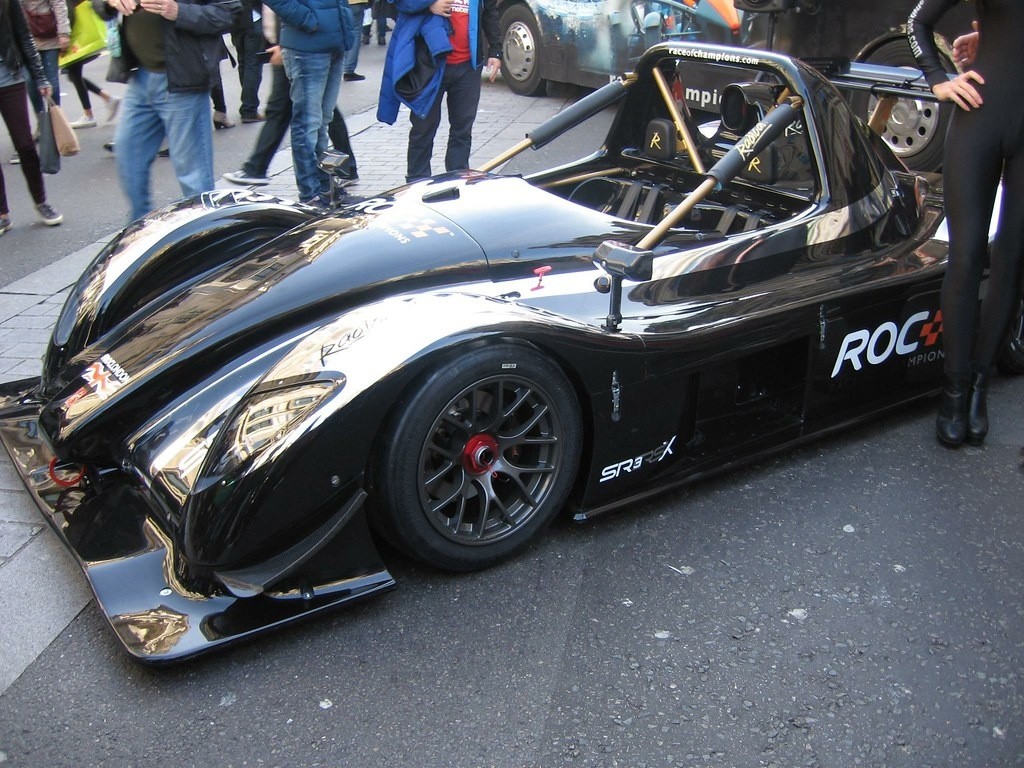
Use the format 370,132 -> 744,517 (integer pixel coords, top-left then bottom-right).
935,360 -> 990,448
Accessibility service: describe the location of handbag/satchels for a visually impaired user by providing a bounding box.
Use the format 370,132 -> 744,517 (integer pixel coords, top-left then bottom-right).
38,94 -> 61,174
47,94 -> 81,157
58,0 -> 109,69
22,2 -> 59,39
105,10 -> 132,83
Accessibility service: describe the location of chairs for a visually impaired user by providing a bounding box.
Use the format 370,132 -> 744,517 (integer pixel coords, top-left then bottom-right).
568,118 -> 779,235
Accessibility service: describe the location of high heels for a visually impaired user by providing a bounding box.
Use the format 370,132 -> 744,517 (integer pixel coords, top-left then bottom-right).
213,111 -> 235,130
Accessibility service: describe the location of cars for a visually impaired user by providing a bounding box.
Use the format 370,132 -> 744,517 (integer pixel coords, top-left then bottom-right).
478,0 -> 745,98
731,0 -> 979,178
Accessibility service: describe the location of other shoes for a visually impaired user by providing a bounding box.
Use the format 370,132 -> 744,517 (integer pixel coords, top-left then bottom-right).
103,142 -> 115,151
106,95 -> 122,122
157,149 -> 171,156
0,218 -> 13,237
70,115 -> 97,129
299,185 -> 365,210
33,201 -> 63,225
241,112 -> 267,123
386,25 -> 393,31
362,33 -> 373,44
10,150 -> 21,163
378,35 -> 386,45
344,71 -> 365,81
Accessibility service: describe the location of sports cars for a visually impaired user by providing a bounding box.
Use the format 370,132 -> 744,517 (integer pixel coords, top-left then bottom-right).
1,43 -> 1024,662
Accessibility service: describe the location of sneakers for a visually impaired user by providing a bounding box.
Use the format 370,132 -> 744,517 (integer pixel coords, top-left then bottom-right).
333,175 -> 360,188
222,170 -> 269,185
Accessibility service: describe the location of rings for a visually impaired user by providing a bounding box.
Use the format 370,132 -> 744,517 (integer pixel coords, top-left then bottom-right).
159,5 -> 161,9
158,11 -> 162,14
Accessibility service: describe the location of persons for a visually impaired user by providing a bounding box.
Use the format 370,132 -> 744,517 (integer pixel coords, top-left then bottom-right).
905,0 -> 1024,448
0,0 -> 400,164
0,0 -> 63,236
90,0 -> 244,226
262,0 -> 366,210
376,0 -> 504,184
221,6 -> 361,189
